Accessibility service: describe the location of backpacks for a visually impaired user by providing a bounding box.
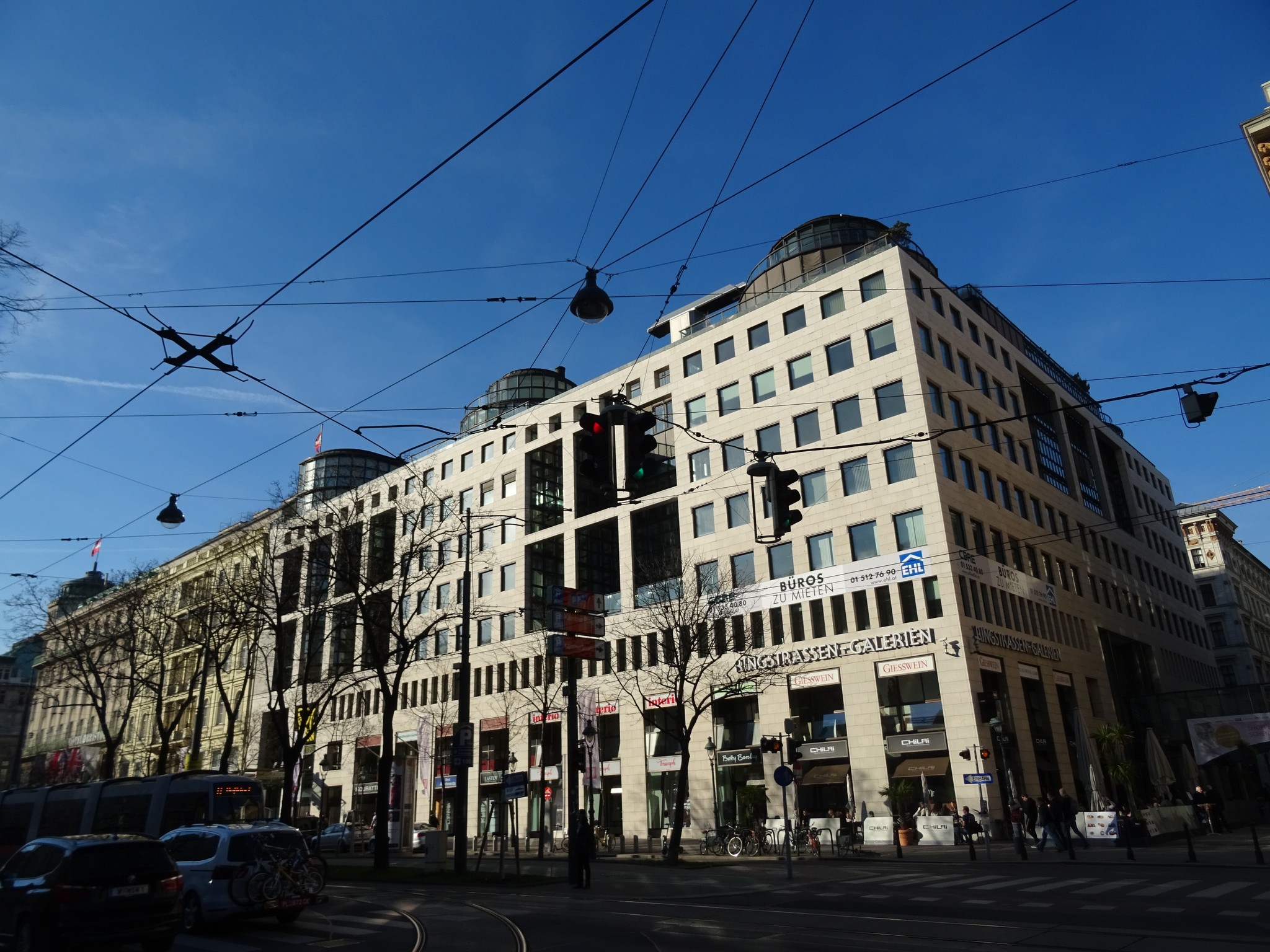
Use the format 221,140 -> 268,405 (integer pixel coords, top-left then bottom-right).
1067,796 -> 1079,815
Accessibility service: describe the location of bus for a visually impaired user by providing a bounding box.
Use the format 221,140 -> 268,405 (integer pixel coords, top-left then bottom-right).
0,770 -> 262,840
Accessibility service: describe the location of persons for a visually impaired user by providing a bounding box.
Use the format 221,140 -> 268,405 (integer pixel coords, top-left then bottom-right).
1153,797 -> 1157,804
1192,786 -> 1232,835
575,809 -> 595,890
1008,788 -> 1090,861
1122,803 -> 1142,830
912,800 -> 976,861
802,807 -> 843,825
429,811 -> 439,828
370,812 -> 377,835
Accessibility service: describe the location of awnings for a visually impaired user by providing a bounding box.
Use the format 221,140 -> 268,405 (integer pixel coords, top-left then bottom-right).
891,757 -> 950,777
801,764 -> 850,786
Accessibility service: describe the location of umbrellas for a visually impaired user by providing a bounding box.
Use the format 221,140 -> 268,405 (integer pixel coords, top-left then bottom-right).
1145,726 -> 1176,805
1074,706 -> 1104,807
1008,768 -> 1021,807
846,773 -> 856,834
1182,743 -> 1213,831
920,771 -> 931,816
1089,764 -> 1107,811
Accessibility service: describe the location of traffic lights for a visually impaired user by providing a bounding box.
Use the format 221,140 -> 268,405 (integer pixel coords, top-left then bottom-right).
959,750 -> 970,761
547,610 -> 605,638
624,410 -> 658,493
786,738 -> 802,763
579,414 -> 611,495
980,749 -> 989,760
759,737 -> 783,754
749,464 -> 802,537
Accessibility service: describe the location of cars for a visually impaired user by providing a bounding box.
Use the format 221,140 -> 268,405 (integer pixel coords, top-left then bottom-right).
413,821 -> 437,854
311,822 -> 373,853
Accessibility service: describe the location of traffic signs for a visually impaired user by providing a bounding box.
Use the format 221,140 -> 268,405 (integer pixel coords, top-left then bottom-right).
547,635 -> 606,660
964,773 -> 994,784
547,585 -> 605,614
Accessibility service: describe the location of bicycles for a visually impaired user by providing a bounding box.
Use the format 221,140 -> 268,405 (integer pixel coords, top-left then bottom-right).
248,838 -> 325,903
780,822 -> 821,858
562,824 -> 616,854
839,836 -> 881,858
699,823 -> 773,856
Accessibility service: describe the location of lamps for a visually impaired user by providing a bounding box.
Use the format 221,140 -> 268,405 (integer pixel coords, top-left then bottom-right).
569,269 -> 614,325
157,494 -> 185,530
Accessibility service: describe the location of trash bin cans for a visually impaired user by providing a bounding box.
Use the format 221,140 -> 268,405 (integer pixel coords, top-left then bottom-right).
425,830 -> 448,862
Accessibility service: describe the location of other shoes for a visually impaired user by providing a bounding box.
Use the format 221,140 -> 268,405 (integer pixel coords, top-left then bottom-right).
1031,840 -> 1041,848
965,840 -> 969,844
1083,843 -> 1090,850
574,884 -> 583,889
584,881 -> 590,888
959,840 -> 965,844
1055,847 -> 1068,852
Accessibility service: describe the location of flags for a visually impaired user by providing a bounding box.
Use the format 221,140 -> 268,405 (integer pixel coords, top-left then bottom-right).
91,538 -> 101,556
315,432 -> 321,453
44,746 -> 100,779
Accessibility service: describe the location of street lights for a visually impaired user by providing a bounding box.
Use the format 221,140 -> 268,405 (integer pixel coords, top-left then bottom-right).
508,751 -> 515,847
989,718 -> 1026,853
358,766 -> 368,810
317,754 -> 330,858
704,736 -> 721,837
582,719 -> 596,861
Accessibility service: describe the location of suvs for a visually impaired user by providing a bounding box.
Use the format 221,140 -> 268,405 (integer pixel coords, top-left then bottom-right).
1,834 -> 182,952
289,816 -> 319,844
157,821 -> 306,939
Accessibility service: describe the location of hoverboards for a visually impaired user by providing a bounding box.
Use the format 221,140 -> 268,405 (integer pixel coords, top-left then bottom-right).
552,824 -> 563,851
